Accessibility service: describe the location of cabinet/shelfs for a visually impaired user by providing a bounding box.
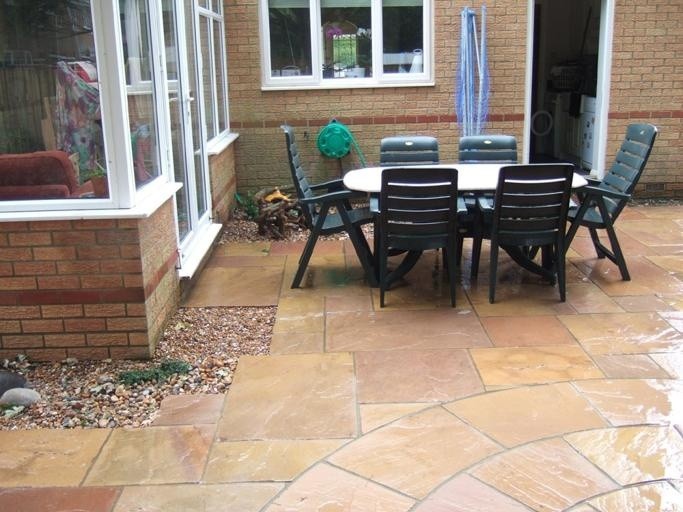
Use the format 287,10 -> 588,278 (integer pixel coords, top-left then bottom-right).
579,96 -> 595,170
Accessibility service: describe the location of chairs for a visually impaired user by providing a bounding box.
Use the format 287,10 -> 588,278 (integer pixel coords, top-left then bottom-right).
0,150 -> 95,201
271,66 -> 301,77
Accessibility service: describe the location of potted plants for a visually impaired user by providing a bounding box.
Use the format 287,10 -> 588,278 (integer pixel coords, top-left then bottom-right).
91,171 -> 108,197
322,64 -> 332,78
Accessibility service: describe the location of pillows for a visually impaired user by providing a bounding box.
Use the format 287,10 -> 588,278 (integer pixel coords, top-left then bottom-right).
0,150 -> 78,194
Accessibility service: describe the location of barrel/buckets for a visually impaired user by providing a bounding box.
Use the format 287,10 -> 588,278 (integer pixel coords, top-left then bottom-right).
409,48 -> 423,73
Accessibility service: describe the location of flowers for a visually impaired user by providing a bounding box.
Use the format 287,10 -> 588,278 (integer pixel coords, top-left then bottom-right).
326,25 -> 371,41
